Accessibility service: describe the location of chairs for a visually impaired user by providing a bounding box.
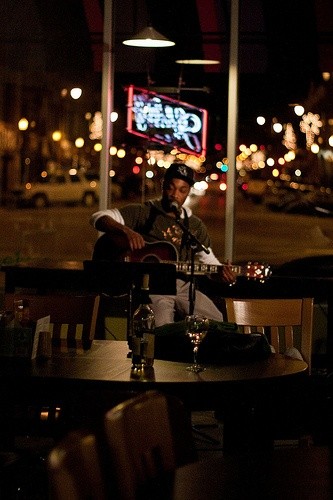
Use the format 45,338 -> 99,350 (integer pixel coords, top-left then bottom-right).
48,295 -> 312,500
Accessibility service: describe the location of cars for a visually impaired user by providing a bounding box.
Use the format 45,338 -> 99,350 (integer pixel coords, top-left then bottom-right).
10,167 -> 122,208
239,167 -> 333,217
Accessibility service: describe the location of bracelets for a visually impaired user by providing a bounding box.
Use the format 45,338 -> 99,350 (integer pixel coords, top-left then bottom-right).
228,280 -> 236,286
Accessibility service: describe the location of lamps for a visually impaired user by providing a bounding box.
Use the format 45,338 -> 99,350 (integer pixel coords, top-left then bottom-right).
122,25 -> 177,48
175,31 -> 220,66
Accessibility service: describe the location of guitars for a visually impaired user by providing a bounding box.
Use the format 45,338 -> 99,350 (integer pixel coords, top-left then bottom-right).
91,230 -> 271,298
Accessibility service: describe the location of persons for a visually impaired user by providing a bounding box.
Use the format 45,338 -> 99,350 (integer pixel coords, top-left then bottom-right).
90,164 -> 256,422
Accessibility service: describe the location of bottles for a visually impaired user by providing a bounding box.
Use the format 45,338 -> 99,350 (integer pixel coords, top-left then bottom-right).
126,273 -> 155,378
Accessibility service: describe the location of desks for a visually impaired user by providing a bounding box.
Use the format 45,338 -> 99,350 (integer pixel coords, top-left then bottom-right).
0,339 -> 306,399
138,448 -> 333,500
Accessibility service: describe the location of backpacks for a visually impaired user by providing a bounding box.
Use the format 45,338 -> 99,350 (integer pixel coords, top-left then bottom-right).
151,316 -> 274,365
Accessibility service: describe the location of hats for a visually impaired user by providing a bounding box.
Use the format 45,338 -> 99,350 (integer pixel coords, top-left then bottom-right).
163,164 -> 195,186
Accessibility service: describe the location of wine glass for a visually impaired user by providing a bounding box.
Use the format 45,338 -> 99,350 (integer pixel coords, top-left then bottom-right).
186,315 -> 209,372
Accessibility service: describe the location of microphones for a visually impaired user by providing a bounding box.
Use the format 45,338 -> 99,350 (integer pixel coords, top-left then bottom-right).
172,202 -> 180,221
